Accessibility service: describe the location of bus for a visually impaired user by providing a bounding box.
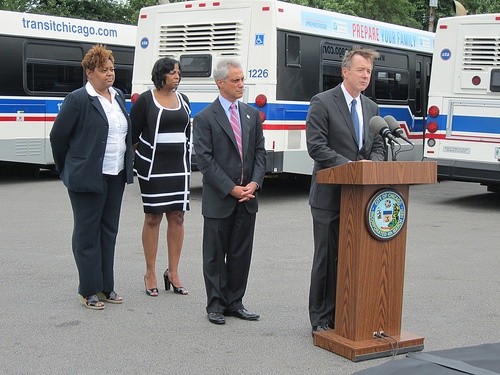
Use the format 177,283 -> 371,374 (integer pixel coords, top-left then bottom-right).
420,12 -> 500,196
129,0 -> 436,189
0,9 -> 138,176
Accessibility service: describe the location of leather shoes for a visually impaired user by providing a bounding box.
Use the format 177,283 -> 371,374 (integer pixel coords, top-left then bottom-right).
312,324 -> 332,337
208,312 -> 226,323
223,307 -> 260,321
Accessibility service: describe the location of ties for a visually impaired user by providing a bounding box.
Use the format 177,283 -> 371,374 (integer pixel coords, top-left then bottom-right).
349,99 -> 360,146
229,103 -> 243,185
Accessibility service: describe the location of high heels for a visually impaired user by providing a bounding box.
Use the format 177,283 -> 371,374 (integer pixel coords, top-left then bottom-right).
97,290 -> 123,303
80,293 -> 106,310
144,274 -> 158,297
164,269 -> 189,295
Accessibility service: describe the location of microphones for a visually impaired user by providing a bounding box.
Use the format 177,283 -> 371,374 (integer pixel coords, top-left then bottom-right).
383,115 -> 409,142
369,116 -> 399,144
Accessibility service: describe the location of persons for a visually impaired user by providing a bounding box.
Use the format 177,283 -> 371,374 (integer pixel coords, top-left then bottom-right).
193,58 -> 266,324
49,44 -> 135,309
304,50 -> 387,338
129,58 -> 191,295
